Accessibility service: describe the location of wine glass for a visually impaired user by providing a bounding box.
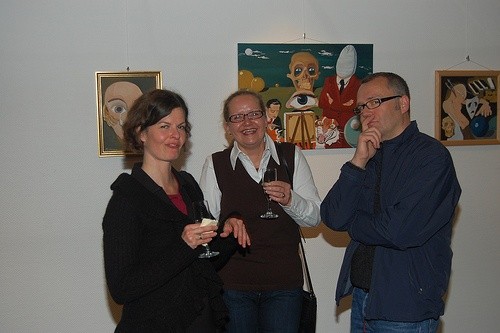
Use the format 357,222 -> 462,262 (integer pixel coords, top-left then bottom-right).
192,199 -> 219,259
260,167 -> 279,219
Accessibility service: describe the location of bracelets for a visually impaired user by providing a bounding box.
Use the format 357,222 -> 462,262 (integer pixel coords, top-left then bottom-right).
279,191 -> 291,206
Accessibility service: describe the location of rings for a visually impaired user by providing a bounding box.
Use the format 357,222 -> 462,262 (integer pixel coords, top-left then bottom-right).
199,234 -> 202,239
282,193 -> 285,197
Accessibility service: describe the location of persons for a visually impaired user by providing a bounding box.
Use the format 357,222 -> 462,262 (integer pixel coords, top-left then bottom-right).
199,91 -> 322,333
320,72 -> 462,333
102,89 -> 251,333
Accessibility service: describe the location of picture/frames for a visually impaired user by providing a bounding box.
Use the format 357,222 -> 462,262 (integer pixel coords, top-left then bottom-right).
95,70 -> 162,158
434,70 -> 500,147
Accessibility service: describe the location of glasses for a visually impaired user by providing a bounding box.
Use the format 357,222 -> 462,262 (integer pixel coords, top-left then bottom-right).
353,95 -> 402,115
227,110 -> 265,123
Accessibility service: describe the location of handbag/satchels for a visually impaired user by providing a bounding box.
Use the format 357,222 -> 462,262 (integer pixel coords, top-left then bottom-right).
299,288 -> 317,333
